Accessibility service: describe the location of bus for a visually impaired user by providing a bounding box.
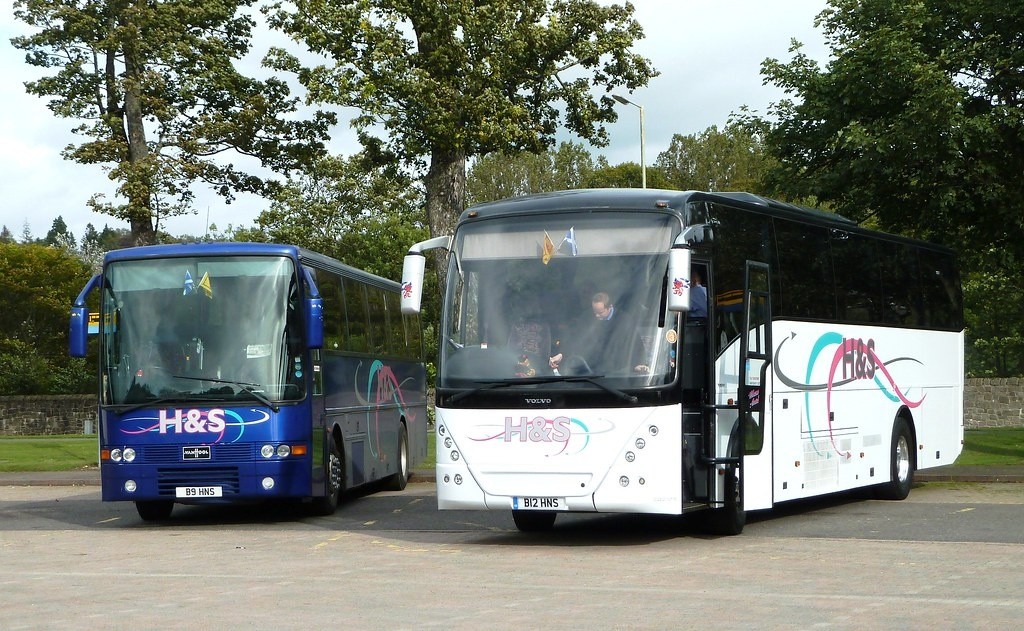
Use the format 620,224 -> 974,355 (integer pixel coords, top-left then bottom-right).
400,186 -> 967,536
68,241 -> 430,523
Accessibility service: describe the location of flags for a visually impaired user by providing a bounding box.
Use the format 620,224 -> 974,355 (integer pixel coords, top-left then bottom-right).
558,227 -> 579,257
198,272 -> 213,299
542,231 -> 555,267
183,271 -> 198,297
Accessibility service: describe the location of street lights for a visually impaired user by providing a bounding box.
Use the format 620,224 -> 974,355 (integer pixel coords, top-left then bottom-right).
612,94 -> 647,188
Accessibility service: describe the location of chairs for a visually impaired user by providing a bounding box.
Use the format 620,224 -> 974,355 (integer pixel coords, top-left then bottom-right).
505,316 -> 556,373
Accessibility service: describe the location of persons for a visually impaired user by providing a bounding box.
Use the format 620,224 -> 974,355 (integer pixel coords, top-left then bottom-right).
688,272 -> 709,327
548,293 -> 653,382
